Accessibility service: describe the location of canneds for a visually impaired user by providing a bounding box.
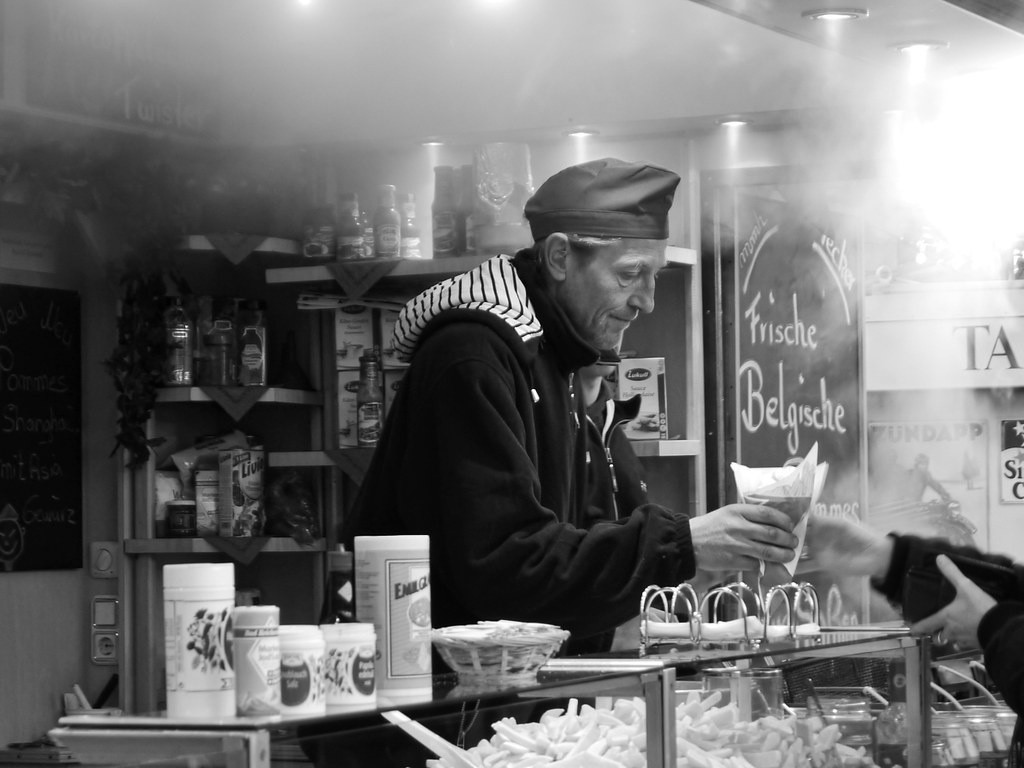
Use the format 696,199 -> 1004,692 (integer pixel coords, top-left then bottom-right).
165,500 -> 196,538
200,333 -> 237,386
300,210 -> 378,264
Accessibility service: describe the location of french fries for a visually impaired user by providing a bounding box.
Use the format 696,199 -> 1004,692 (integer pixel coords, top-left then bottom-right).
427,691 -> 881,768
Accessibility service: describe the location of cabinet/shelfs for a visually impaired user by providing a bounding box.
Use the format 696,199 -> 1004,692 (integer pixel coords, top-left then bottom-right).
121,231 -> 706,716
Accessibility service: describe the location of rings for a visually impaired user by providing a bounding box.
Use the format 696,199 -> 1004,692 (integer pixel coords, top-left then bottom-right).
937,631 -> 948,646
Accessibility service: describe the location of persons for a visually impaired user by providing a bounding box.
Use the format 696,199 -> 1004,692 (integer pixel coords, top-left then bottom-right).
802,511 -> 1024,768
348,159 -> 798,660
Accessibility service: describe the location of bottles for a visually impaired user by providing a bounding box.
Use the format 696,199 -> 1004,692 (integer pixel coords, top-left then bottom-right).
299,163 -> 475,260
161,532 -> 433,720
152,268 -> 269,387
698,667 -> 1017,768
165,500 -> 195,538
356,355 -> 384,448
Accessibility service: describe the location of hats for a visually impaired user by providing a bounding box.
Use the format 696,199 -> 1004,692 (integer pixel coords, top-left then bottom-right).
525,158 -> 681,241
